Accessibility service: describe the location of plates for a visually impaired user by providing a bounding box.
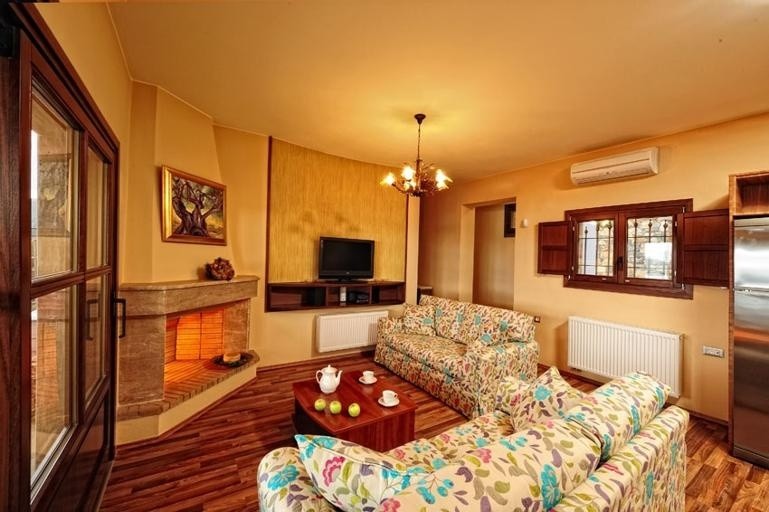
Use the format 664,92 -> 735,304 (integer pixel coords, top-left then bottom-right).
378,396 -> 400,407
358,376 -> 378,384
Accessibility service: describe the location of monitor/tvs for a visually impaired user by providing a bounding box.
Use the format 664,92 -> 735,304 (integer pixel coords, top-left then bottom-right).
318,237 -> 375,284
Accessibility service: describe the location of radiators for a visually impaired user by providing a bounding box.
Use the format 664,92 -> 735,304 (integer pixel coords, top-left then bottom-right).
565,316 -> 686,402
314,309 -> 389,354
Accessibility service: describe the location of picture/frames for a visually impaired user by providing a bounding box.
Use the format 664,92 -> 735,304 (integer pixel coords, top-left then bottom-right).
159,164 -> 227,249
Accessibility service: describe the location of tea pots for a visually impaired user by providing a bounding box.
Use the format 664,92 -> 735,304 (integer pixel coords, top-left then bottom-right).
315,364 -> 343,394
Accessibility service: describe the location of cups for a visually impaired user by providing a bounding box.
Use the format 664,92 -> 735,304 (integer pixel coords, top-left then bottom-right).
362,371 -> 374,381
382,390 -> 398,404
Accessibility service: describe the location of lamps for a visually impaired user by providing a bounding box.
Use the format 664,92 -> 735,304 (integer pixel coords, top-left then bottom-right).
381,113 -> 451,198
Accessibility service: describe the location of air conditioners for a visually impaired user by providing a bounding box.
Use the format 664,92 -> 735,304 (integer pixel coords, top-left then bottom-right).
571,147 -> 661,187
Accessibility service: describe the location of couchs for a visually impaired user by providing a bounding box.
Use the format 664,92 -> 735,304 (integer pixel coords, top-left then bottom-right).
374,292 -> 540,420
255,363 -> 692,512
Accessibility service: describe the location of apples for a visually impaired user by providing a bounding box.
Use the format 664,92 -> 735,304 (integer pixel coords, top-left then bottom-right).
314,399 -> 326,411
329,400 -> 342,414
348,403 -> 360,417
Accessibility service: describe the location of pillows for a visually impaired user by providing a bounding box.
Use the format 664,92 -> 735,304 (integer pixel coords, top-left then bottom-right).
465,321 -> 509,357
401,301 -> 434,335
293,433 -> 416,512
503,366 -> 587,433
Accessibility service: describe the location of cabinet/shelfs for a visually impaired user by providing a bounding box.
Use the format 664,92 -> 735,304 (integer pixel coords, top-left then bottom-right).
267,281 -> 406,310
730,170 -> 769,467
